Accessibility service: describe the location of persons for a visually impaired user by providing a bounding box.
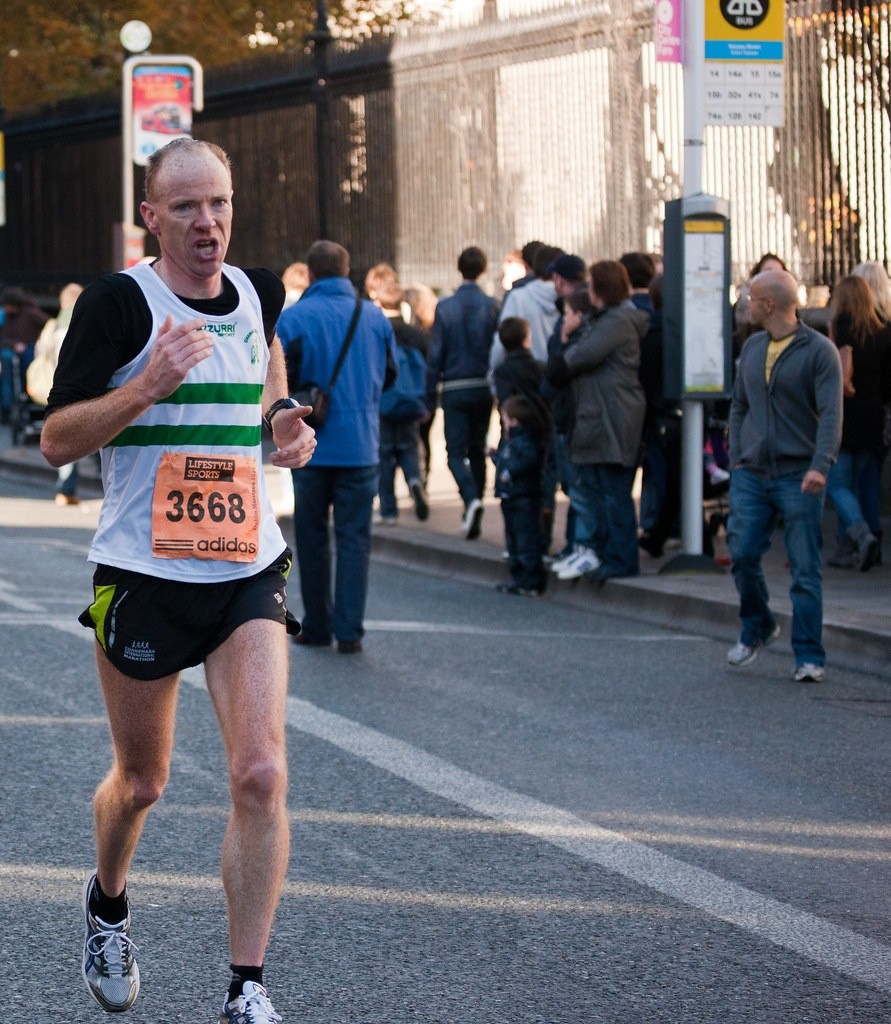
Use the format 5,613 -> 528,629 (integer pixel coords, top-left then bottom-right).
719,268 -> 843,684
280,263 -> 310,313
41,138 -> 319,1024
367,242 -> 891,598
274,239 -> 412,654
27,279 -> 86,506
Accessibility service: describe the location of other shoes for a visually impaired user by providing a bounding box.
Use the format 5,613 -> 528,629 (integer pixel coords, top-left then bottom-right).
290,628 -> 332,645
638,532 -> 663,558
583,565 -> 616,580
852,534 -> 878,571
374,513 -> 396,526
408,480 -> 430,521
827,549 -> 855,567
338,638 -> 363,653
464,498 -> 485,539
55,491 -> 81,505
496,582 -> 539,597
711,468 -> 731,485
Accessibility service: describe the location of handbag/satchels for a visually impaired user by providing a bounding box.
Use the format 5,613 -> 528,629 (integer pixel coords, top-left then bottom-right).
288,385 -> 328,429
26,356 -> 56,407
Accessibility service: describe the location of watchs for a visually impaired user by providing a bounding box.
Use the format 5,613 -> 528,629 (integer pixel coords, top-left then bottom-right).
262,397 -> 302,436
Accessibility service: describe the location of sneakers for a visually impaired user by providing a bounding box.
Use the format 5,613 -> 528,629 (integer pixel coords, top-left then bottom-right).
557,548 -> 602,579
218,980 -> 283,1024
551,545 -> 586,572
82,864 -> 141,1010
794,662 -> 826,683
727,623 -> 780,667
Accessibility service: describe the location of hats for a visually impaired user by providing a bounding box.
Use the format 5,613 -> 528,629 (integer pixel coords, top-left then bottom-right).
548,254 -> 586,281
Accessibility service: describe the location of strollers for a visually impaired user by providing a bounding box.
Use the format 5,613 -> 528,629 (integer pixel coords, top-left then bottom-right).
10,343 -> 52,451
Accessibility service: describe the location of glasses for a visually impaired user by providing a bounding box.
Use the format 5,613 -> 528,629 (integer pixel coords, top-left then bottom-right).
746,293 -> 767,305
